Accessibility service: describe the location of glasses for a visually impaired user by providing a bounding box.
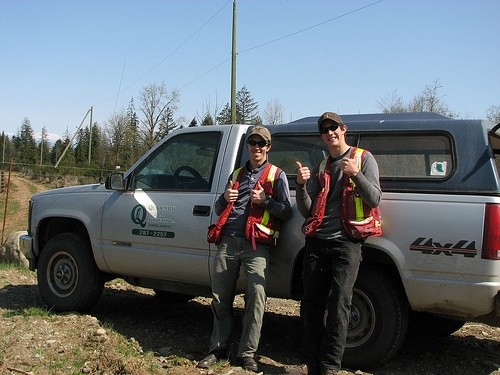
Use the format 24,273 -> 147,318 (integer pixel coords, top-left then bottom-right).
319,122 -> 341,134
246,140 -> 267,147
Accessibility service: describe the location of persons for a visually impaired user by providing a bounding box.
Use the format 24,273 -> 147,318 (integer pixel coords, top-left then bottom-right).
285,112 -> 383,375
198,126 -> 293,372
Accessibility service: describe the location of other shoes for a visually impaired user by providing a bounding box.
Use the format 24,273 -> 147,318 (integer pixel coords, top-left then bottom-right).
239,357 -> 258,371
198,353 -> 217,368
323,368 -> 340,375
305,360 -> 322,375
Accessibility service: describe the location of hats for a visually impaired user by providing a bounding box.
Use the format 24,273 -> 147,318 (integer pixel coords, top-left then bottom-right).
246,126 -> 272,143
318,112 -> 343,127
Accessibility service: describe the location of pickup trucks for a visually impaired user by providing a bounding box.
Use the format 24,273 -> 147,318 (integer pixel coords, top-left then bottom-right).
17,109 -> 500,371
